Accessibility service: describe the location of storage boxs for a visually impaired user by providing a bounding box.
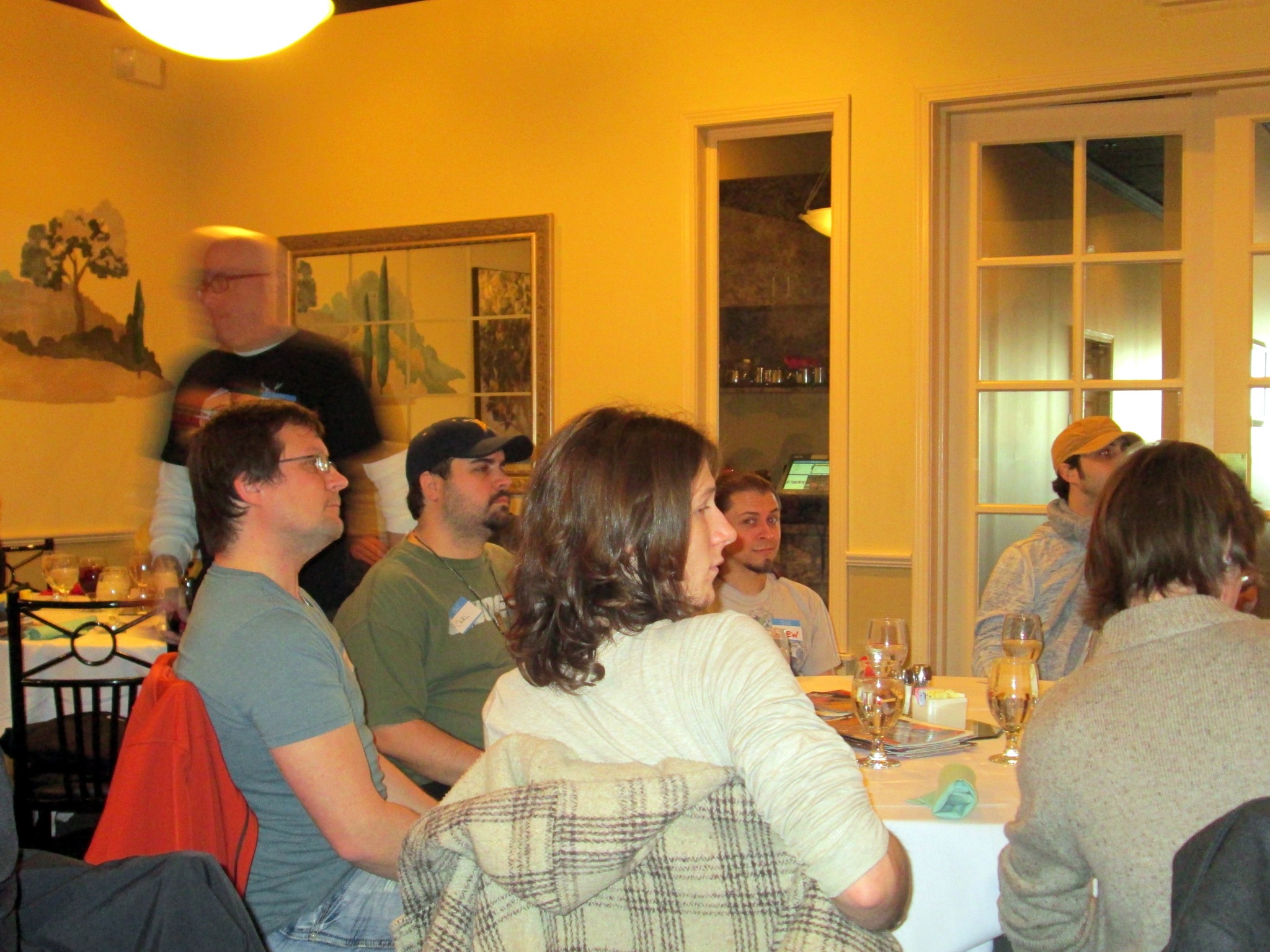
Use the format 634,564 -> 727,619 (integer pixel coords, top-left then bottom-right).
911,697 -> 969,729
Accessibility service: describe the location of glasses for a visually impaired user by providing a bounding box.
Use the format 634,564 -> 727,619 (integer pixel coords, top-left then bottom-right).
193,271 -> 272,301
278,454 -> 337,474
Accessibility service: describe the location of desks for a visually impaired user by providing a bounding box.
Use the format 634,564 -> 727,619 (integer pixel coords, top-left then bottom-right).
796,672 -> 1059,952
0,585 -> 169,734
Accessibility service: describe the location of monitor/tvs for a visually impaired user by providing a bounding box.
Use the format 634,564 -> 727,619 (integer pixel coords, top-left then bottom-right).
775,454 -> 830,498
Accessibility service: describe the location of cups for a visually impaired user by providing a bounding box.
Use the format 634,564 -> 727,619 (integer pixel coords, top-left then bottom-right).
725,358 -> 827,388
766,626 -> 791,665
867,618 -> 908,666
1001,613 -> 1043,662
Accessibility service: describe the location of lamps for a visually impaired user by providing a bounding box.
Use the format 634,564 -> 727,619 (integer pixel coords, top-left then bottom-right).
797,152 -> 831,236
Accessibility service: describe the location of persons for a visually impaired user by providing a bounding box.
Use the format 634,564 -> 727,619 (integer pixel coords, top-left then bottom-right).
174,401 -> 438,952
997,438 -> 1270,952
972,415 -> 1257,681
137,224 -> 416,655
332,414 -> 534,798
711,469 -> 843,677
480,407 -> 915,931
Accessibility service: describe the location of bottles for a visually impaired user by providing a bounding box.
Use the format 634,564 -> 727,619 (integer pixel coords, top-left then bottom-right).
912,664 -> 932,688
901,667 -> 913,717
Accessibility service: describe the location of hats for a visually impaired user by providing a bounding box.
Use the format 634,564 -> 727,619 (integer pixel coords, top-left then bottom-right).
406,416 -> 535,483
1052,416 -> 1146,474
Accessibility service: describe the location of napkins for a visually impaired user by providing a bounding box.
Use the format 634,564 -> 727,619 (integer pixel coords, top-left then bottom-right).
909,761 -> 978,820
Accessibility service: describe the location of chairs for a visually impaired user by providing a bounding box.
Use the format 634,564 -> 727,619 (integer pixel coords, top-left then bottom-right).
0,538 -> 270,952
390,729 -> 904,952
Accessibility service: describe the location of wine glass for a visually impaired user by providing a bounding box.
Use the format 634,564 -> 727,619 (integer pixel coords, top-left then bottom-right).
850,658 -> 906,770
42,553 -> 79,602
95,565 -> 131,634
986,657 -> 1040,765
126,548 -> 152,615
78,556 -> 104,613
